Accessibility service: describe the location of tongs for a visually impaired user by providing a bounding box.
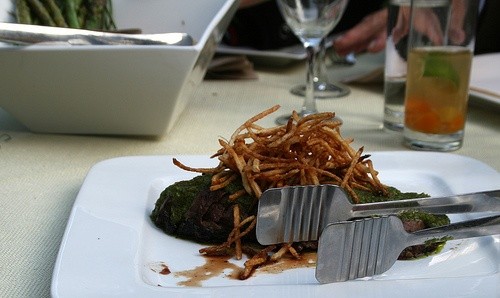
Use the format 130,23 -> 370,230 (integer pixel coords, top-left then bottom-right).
256,183 -> 500,284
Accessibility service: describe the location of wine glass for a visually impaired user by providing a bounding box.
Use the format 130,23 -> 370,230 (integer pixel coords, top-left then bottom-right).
290,30 -> 351,99
274,0 -> 349,130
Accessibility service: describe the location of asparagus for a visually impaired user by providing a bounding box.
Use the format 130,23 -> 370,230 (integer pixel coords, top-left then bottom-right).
13,0 -> 115,33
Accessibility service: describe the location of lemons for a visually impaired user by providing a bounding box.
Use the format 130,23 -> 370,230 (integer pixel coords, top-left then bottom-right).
422,57 -> 460,94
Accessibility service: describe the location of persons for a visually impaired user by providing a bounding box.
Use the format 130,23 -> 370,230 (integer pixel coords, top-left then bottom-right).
332,0 -> 500,57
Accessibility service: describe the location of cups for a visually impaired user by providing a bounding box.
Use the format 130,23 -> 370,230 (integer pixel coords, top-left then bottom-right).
400,0 -> 478,152
383,0 -> 453,132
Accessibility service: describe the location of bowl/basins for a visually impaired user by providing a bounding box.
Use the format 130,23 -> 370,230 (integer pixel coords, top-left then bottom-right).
468,53 -> 500,111
0,0 -> 240,136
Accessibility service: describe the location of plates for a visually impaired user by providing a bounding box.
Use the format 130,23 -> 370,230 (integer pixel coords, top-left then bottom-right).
49,150 -> 500,297
214,35 -> 343,68
